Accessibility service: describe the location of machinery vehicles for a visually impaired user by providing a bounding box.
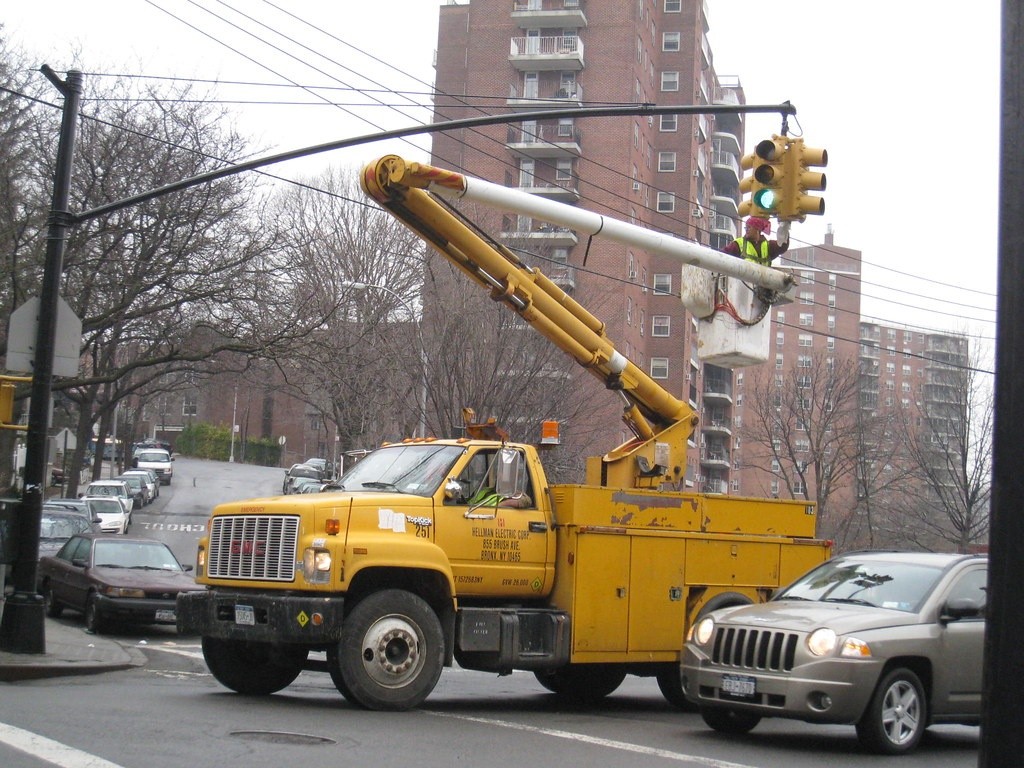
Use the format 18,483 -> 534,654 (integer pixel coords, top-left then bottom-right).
173,155 -> 832,712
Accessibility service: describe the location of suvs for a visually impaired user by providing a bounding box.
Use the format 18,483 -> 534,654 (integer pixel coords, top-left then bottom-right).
87,479 -> 134,524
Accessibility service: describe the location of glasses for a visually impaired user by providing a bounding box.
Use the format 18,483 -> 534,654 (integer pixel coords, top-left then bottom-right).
745,227 -> 754,231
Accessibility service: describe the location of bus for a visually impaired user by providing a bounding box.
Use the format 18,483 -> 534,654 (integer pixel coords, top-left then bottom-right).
89,438 -> 125,462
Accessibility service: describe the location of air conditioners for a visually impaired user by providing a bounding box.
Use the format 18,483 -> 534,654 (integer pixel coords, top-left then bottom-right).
631,271 -> 637,278
693,169 -> 699,178
709,211 -> 715,218
633,183 -> 639,191
699,442 -> 706,449
686,374 -> 691,381
710,114 -> 716,121
696,89 -> 701,98
692,209 -> 701,218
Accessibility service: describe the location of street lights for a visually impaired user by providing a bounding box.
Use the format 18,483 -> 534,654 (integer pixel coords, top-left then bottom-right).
208,359 -> 240,463
342,282 -> 430,440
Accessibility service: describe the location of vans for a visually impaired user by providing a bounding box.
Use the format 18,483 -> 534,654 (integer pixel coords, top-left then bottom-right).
132,447 -> 175,487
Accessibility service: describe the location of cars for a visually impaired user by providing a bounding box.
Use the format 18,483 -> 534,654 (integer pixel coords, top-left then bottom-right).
678,549 -> 988,761
37,533 -> 209,635
38,500 -> 104,555
116,468 -> 160,508
78,495 -> 129,537
279,456 -> 347,495
51,467 -> 64,486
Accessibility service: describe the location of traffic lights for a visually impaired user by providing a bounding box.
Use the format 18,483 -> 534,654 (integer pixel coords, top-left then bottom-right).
750,138 -> 790,219
791,137 -> 829,221
737,153 -> 752,218
0,374 -> 33,431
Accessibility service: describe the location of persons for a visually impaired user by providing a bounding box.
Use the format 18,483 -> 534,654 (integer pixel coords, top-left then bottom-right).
456,464 -> 532,509
724,216 -> 791,267
